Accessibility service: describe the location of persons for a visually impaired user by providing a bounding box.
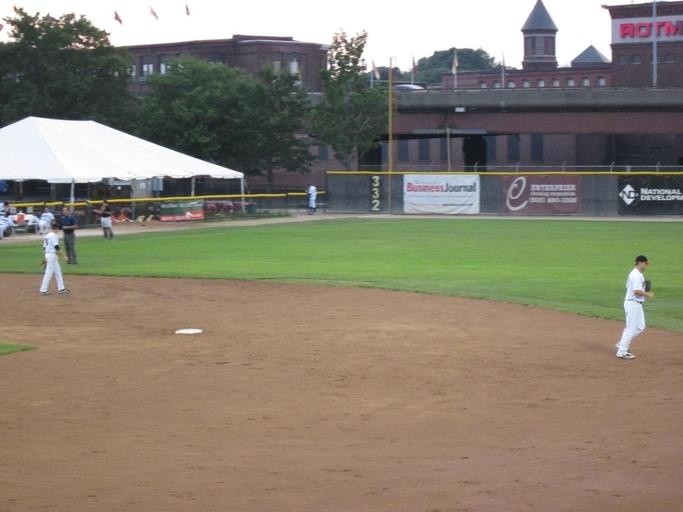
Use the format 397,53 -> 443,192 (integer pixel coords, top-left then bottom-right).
616,253 -> 655,361
36,220 -> 70,294
0,199 -> 161,241
57,206 -> 79,265
183,207 -> 203,221
306,183 -> 318,215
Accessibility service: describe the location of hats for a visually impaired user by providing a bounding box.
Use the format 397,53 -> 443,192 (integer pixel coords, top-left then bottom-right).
635,254 -> 653,264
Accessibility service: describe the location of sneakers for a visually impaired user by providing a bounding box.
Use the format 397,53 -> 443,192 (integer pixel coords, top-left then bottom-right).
39,291 -> 54,295
614,341 -> 636,359
58,288 -> 73,294
